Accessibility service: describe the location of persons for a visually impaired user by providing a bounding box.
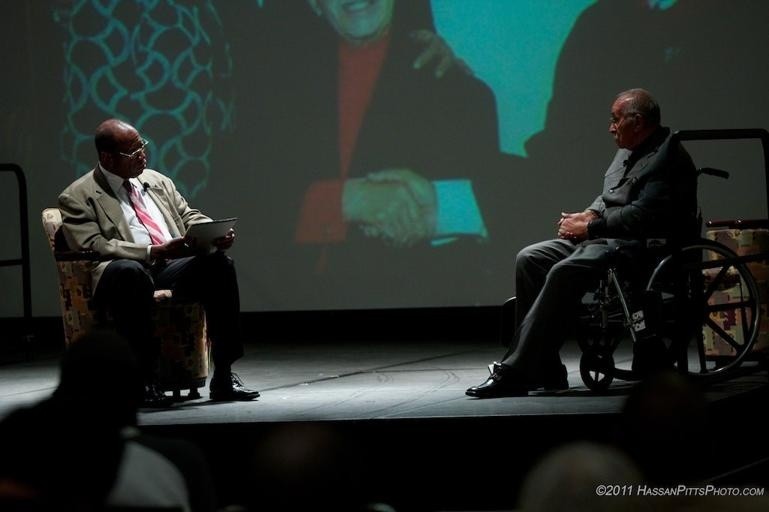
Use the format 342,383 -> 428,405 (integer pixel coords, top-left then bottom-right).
356,0 -> 768,309
202,0 -> 473,107
0,325 -> 192,509
190,1 -> 498,315
56,117 -> 262,410
465,86 -> 701,400
521,439 -> 647,512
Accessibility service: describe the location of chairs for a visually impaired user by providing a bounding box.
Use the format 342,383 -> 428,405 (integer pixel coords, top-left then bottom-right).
701,218 -> 769,372
40,206 -> 215,401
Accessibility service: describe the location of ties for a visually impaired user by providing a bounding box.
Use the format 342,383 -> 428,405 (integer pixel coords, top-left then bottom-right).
122,180 -> 166,245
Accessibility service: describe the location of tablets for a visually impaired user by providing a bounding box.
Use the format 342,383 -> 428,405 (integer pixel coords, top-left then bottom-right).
183,218 -> 239,254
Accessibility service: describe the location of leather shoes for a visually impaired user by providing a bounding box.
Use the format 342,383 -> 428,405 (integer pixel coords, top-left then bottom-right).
465,364 -> 570,397
137,385 -> 175,411
209,376 -> 260,401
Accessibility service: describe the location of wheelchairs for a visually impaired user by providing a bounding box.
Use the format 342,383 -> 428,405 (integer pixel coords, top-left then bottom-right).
576,166 -> 762,394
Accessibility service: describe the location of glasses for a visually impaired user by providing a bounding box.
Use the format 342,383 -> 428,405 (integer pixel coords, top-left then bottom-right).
114,137 -> 149,159
